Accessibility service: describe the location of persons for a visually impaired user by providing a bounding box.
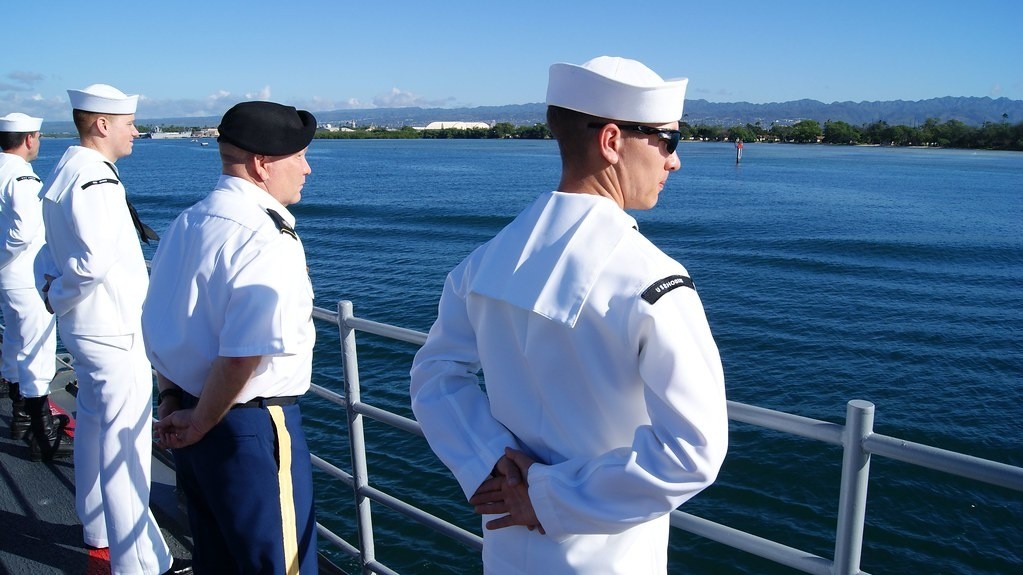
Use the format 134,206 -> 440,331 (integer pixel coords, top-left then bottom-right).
0,112 -> 75,463
33,84 -> 193,575
408,54 -> 731,575
140,100 -> 319,574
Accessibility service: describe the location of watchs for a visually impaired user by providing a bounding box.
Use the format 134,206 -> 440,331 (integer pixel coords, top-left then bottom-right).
157,388 -> 183,407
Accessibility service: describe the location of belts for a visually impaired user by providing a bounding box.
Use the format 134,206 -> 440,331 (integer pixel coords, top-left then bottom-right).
215,396 -> 303,408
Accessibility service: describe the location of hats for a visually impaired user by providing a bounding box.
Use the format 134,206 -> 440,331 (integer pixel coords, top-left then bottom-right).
0,112 -> 42,132
67,83 -> 138,114
546,54 -> 690,123
216,101 -> 316,155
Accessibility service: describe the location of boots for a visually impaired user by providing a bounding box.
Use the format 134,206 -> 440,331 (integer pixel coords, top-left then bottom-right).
6,380 -> 69,439
26,395 -> 80,463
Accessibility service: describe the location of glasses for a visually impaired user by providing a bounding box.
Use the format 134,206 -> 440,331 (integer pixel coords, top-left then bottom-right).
586,120 -> 681,154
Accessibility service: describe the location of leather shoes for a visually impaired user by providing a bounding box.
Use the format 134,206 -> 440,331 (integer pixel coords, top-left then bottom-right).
162,552 -> 192,575
